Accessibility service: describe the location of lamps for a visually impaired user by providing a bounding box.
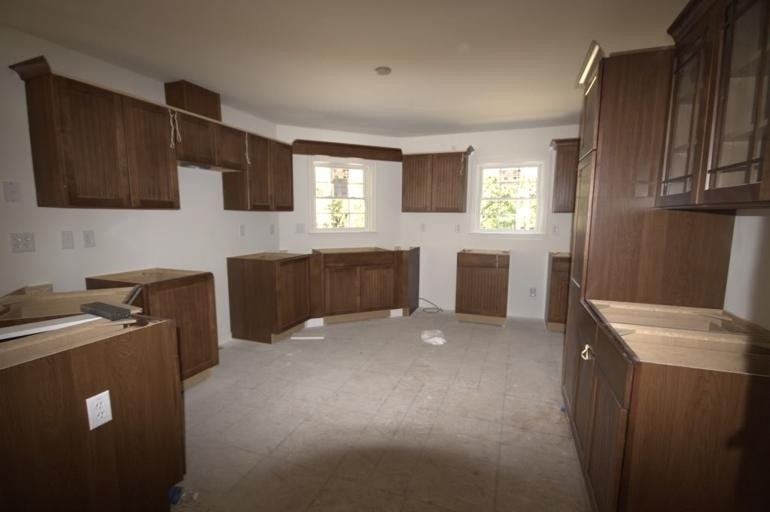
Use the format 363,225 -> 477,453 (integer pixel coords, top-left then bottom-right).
576,40 -> 605,90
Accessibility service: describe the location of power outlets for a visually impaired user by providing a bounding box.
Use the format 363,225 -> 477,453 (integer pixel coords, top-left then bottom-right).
83,393 -> 112,431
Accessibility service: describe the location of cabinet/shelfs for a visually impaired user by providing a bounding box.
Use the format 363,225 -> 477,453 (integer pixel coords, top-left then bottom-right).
455,247 -> 510,317
1,283 -> 187,512
9,53 -> 181,211
401,145 -> 474,213
550,137 -> 579,212
172,105 -> 245,173
228,252 -> 312,343
570,301 -> 769,509
658,3 -> 770,212
221,131 -> 292,213
312,246 -> 420,322
85,267 -> 219,378
561,45 -> 736,421
543,251 -> 571,332
163,80 -> 221,122
